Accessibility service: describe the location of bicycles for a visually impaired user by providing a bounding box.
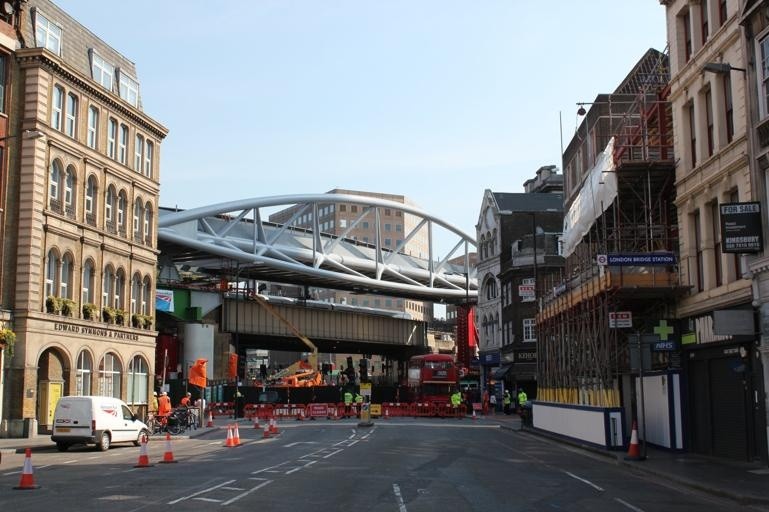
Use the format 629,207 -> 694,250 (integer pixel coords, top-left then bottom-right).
144,408 -> 197,435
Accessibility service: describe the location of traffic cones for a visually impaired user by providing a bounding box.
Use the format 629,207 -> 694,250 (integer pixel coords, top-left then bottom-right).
252,414 -> 279,439
223,421 -> 242,448
133,433 -> 153,466
13,448 -> 40,490
625,420 -> 647,462
158,432 -> 176,463
471,409 -> 479,419
295,408 -> 389,422
206,411 -> 214,427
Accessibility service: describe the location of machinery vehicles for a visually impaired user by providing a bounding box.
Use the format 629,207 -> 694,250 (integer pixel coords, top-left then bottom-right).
243,290 -> 327,387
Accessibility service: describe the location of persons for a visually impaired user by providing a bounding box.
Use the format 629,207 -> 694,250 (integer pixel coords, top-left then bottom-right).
194,393 -> 206,428
354,391 -> 363,419
503,390 -> 512,416
158,391 -> 171,416
450,388 -> 464,418
340,388 -> 353,419
517,387 -> 528,418
180,392 -> 192,407
489,393 -> 497,417
153,391 -> 159,415
483,390 -> 490,417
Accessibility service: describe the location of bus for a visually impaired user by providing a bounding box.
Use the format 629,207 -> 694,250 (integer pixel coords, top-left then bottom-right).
407,352 -> 458,408
458,363 -> 480,391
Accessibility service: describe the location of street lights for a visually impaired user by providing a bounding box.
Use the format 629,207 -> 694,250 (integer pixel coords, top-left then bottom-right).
494,210 -> 540,309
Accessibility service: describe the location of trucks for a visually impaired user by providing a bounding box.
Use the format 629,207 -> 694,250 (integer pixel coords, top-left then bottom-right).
50,396 -> 151,452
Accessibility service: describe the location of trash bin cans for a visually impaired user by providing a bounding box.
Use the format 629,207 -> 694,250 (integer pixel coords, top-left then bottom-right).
23,418 -> 38,438
